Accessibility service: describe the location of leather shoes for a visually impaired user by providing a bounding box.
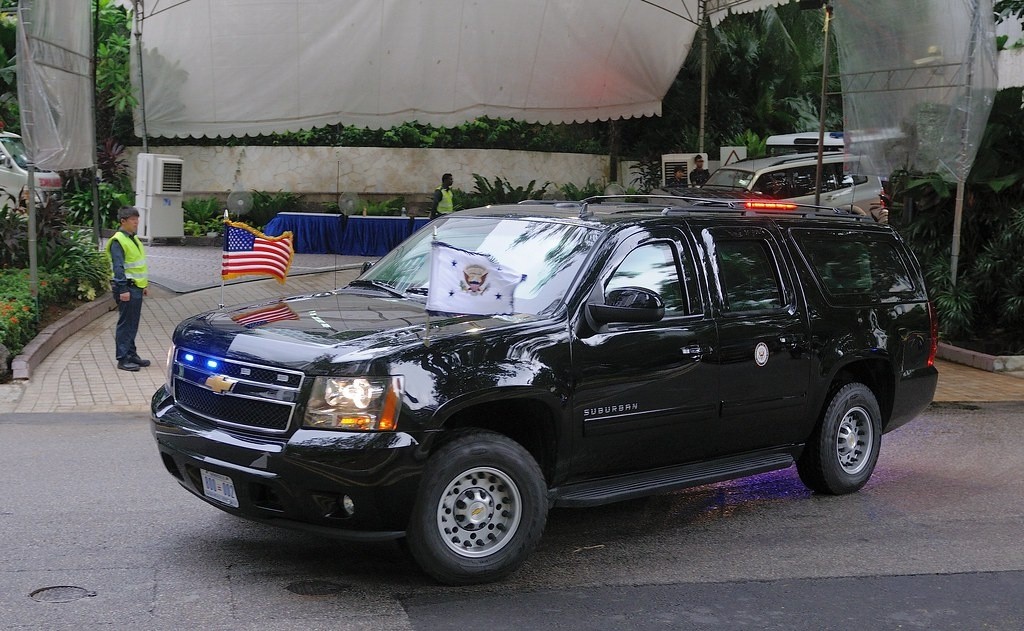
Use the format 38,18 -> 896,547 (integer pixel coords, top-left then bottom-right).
129,355 -> 150,366
117,358 -> 139,370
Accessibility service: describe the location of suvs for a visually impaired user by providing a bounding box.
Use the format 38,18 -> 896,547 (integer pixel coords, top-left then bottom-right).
150,195 -> 940,585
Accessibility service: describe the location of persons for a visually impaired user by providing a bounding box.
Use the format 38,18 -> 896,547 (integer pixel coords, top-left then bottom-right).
106,206 -> 151,371
429,173 -> 455,221
689,155 -> 711,188
667,165 -> 689,188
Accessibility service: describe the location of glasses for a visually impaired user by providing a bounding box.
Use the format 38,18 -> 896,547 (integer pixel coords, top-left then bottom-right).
697,160 -> 704,162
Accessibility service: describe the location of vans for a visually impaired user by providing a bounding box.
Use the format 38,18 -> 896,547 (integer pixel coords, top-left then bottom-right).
698,150 -> 890,225
0,120 -> 65,222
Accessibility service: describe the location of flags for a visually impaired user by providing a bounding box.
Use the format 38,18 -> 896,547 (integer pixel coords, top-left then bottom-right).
230,301 -> 299,329
425,240 -> 527,316
221,216 -> 294,284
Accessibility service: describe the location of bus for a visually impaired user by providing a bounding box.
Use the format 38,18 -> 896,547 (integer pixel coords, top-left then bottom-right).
765,123 -> 918,191
